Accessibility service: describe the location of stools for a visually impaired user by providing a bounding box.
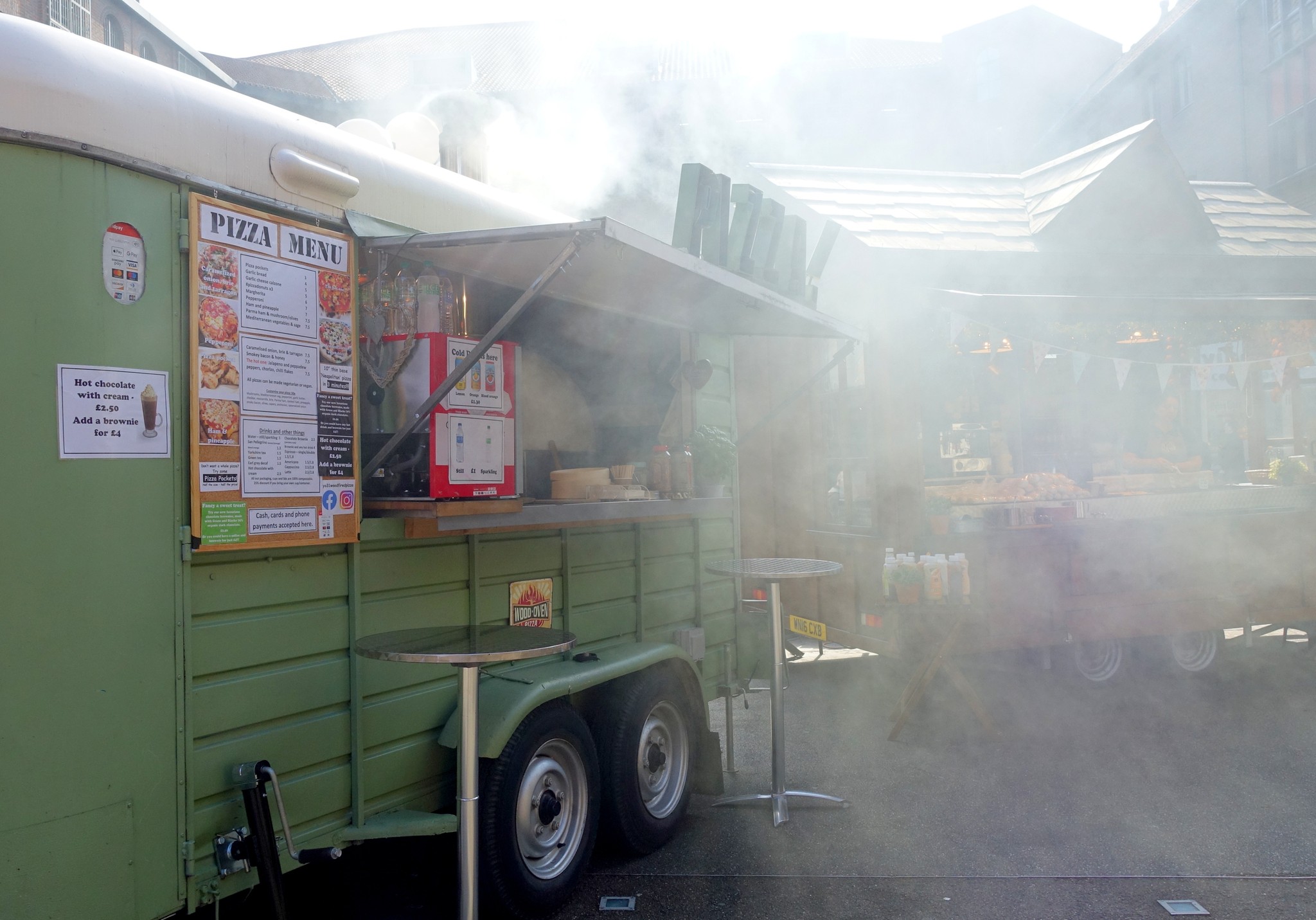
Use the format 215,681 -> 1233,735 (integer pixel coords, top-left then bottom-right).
702,556 -> 853,830
348,622 -> 581,920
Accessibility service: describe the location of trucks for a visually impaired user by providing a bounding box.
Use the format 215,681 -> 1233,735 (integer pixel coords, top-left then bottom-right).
1,8 -> 857,920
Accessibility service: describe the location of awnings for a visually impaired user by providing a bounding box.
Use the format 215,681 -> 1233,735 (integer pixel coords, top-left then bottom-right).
360,215 -> 871,486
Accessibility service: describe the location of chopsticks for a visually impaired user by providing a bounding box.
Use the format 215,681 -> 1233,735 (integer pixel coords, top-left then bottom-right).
610,465 -> 634,478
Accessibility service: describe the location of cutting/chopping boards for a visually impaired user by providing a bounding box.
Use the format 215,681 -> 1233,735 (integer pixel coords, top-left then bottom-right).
200,397 -> 240,445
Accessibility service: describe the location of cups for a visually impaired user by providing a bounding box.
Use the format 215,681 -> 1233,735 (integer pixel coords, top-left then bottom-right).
141,395 -> 163,438
613,478 -> 633,490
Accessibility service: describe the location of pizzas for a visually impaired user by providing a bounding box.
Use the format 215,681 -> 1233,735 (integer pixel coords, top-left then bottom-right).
318,271 -> 351,314
198,297 -> 238,350
197,245 -> 238,298
200,399 -> 240,439
318,321 -> 352,364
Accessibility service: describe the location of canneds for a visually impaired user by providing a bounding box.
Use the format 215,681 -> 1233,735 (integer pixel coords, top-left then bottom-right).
485,361 -> 496,393
471,361 -> 481,392
455,357 -> 466,390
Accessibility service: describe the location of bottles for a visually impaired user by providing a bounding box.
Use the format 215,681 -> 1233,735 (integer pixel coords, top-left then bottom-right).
885,548 -> 895,557
417,261 -> 440,333
456,423 -> 464,463
486,426 -> 491,463
485,361 -> 496,392
908,552 -> 917,564
455,357 -> 467,390
437,270 -> 453,336
373,270 -> 394,336
671,446 -> 694,493
947,555 -> 960,599
900,557 -> 920,604
923,558 -> 943,600
955,553 -> 971,595
896,554 -> 906,566
935,554 -> 949,596
626,462 -> 646,490
393,262 -> 417,335
917,555 -> 931,576
650,445 -> 671,492
471,360 -> 480,392
883,558 -> 898,599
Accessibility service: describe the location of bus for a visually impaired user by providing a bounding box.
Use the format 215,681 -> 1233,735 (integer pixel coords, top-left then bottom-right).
737,286 -> 1316,704
